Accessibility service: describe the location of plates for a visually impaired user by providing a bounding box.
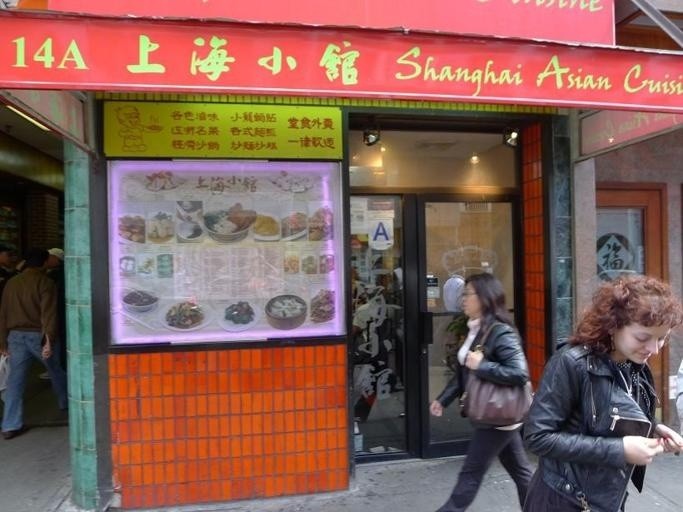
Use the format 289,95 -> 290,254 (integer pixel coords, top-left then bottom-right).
162,303 -> 261,332
116,201 -> 335,276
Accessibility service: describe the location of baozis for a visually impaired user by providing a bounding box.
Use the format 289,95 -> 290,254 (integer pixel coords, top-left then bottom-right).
121,259 -> 134,271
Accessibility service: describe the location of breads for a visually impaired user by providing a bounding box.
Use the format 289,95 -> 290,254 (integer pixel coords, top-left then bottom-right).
282,213 -> 307,238
308,206 -> 333,241
253,214 -> 277,237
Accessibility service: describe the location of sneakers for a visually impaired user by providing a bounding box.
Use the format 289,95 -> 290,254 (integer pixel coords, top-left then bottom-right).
1,424 -> 30,439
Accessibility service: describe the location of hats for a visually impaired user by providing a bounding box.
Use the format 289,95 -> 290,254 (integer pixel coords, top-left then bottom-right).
48,248 -> 66,261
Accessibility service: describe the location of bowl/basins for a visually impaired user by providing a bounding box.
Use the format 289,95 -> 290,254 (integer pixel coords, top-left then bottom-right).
119,288 -> 159,314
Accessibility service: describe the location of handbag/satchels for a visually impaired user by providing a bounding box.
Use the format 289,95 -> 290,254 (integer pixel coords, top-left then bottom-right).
464,370 -> 534,430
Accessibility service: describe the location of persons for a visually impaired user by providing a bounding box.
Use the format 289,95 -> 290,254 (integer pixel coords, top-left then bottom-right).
0,245 -> 67,440
443,265 -> 466,315
523,275 -> 683,511
428,273 -> 534,511
352,253 -> 406,421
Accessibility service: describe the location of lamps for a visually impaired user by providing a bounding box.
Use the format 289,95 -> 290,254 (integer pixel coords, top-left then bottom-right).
502,126 -> 519,148
361,122 -> 380,146
